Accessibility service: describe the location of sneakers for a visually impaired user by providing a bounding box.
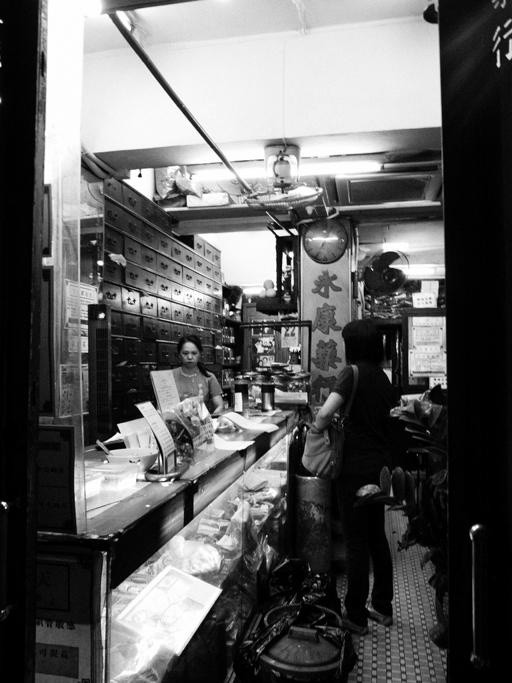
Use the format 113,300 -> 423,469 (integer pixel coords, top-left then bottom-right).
365,599 -> 393,627
341,609 -> 368,636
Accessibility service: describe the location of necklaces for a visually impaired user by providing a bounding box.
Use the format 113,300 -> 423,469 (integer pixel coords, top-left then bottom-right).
179,366 -> 201,377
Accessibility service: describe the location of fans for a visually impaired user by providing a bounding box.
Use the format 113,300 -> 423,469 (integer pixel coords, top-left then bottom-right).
351,245 -> 412,302
241,143 -> 327,213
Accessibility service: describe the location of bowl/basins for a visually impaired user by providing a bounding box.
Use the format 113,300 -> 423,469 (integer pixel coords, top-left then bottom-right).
105,448 -> 159,472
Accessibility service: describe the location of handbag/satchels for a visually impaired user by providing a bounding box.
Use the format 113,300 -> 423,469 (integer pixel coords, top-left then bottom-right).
301,411 -> 345,481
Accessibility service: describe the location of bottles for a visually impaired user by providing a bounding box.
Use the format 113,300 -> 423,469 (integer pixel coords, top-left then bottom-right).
261,383 -> 275,412
235,384 -> 249,414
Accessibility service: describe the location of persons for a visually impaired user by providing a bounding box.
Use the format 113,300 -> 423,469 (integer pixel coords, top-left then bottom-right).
316,317 -> 402,637
170,333 -> 225,417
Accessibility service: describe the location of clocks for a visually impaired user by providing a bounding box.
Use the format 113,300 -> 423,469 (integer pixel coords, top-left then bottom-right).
302,216 -> 349,265
